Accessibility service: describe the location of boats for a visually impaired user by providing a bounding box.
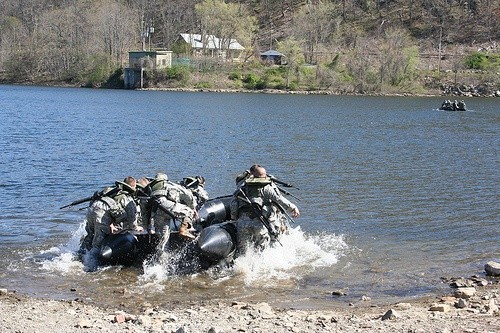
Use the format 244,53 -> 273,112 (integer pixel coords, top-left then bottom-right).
441,102 -> 462,110
100,194 -> 238,269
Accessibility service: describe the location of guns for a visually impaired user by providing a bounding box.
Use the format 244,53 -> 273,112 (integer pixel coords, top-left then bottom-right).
277,186 -> 301,202
233,188 -> 283,248
59,184 -> 123,210
274,200 -> 295,224
267,173 -> 300,191
135,187 -> 186,227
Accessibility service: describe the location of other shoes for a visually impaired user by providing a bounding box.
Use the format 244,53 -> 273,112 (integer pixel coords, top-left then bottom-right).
180,228 -> 195,239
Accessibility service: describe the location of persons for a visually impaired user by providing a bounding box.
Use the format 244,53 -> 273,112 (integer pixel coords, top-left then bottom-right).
235,164 -> 263,188
176,175 -> 209,204
439,98 -> 466,110
234,167 -> 301,260
142,171 -> 198,262
74,175 -> 140,273
